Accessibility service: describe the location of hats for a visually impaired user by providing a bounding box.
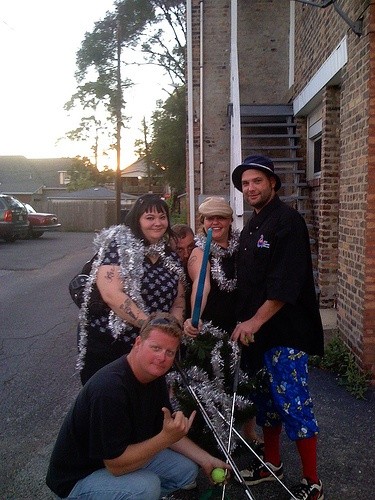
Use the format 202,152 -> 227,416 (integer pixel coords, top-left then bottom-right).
232,156 -> 281,193
199,197 -> 232,219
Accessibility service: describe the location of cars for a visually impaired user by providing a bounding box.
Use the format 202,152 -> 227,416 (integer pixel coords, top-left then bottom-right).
23,201 -> 62,239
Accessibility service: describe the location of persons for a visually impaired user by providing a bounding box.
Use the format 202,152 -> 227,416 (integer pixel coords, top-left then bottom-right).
45,313 -> 234,500
231,156 -> 329,500
75,194 -> 264,455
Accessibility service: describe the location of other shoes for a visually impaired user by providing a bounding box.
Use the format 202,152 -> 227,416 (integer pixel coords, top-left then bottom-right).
182,480 -> 197,490
240,437 -> 265,455
160,494 -> 182,500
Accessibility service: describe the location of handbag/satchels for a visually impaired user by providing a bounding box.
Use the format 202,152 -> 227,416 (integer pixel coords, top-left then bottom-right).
69,247 -> 105,316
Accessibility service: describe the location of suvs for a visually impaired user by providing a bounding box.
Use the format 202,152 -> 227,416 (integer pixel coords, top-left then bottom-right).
0,194 -> 30,242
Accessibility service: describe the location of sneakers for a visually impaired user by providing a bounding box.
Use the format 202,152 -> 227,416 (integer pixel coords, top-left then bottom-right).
290,478 -> 325,500
234,461 -> 284,485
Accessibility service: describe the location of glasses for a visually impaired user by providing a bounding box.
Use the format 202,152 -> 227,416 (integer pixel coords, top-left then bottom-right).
141,318 -> 181,333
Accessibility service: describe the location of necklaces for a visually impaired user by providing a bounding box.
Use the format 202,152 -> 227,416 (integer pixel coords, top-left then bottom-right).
144,242 -> 188,291
195,228 -> 239,290
